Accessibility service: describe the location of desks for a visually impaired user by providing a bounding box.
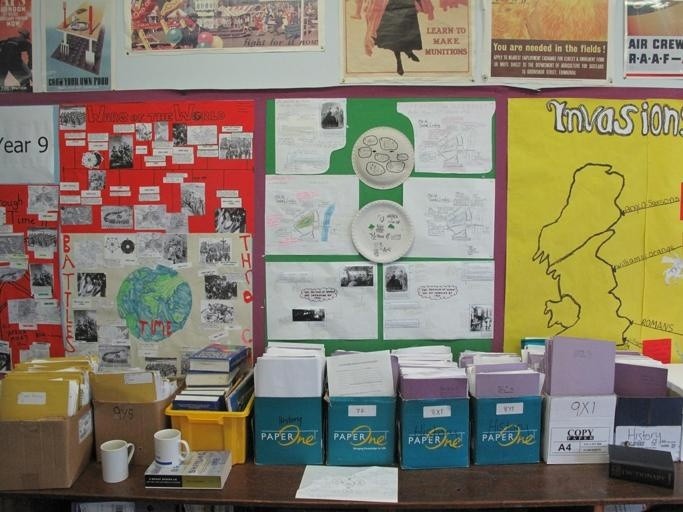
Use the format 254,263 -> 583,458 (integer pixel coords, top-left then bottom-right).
1,457 -> 682,511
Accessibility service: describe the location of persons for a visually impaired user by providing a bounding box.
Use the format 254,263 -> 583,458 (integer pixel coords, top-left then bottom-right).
59,110 -> 86,129
30,267 -> 51,287
27,231 -> 55,248
164,235 -> 186,264
200,239 -> 231,263
203,300 -> 234,323
353,1 -> 443,81
76,271 -> 105,298
73,315 -> 96,342
0,29 -> 31,92
215,209 -> 244,234
218,133 -> 252,160
340,265 -> 372,287
203,273 -> 237,300
385,266 -> 408,292
320,102 -> 343,129
109,142 -> 133,169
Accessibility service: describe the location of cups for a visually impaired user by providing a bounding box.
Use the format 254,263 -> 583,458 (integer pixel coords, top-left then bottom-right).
100,440 -> 137,486
154,428 -> 190,469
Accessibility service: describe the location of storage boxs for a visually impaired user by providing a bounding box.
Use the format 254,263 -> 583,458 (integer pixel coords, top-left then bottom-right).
251,395 -> 683,469
88,378 -> 186,466
163,391 -> 254,466
0,400 -> 97,489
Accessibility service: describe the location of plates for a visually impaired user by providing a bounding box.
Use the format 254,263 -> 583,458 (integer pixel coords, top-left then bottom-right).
351,128 -> 413,188
350,200 -> 415,264
